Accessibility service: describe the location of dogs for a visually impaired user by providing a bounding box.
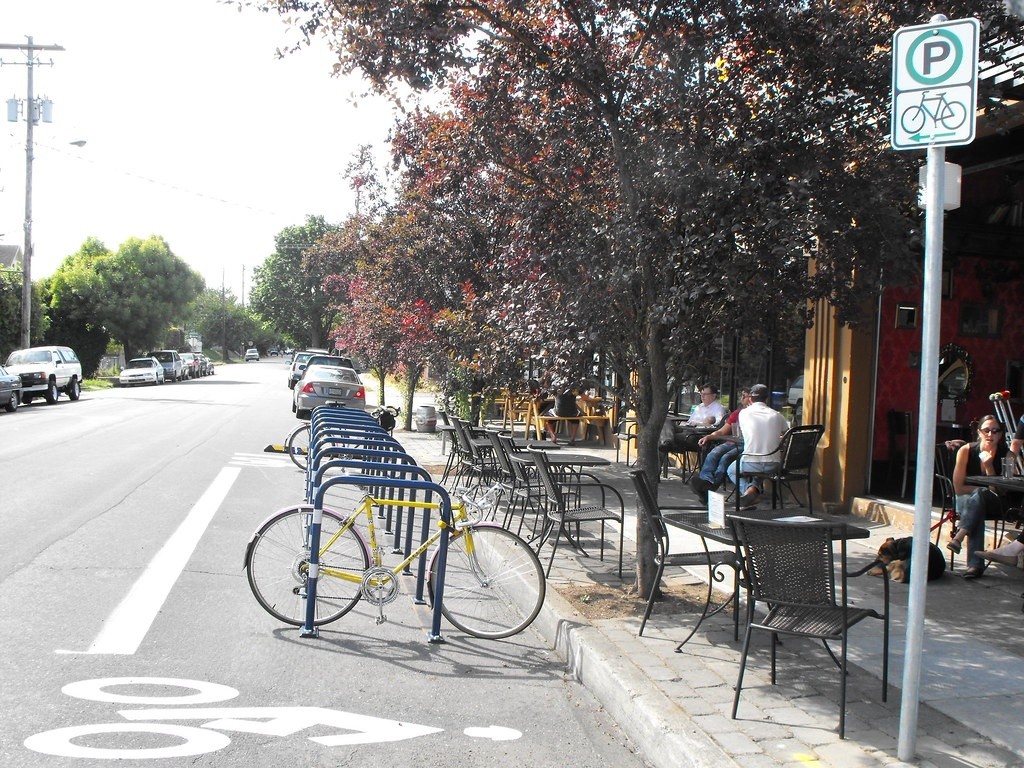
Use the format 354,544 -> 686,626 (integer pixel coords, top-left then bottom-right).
864,536 -> 946,583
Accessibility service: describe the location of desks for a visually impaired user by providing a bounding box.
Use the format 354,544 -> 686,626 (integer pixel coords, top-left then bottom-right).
715,434 -> 804,507
661,507 -> 871,685
675,426 -> 720,484
477,387 -> 604,441
508,453 -> 611,544
937,422 -> 1007,454
626,416 -> 688,471
966,476 -> 1024,571
436,425 -> 512,494
470,439 -> 561,521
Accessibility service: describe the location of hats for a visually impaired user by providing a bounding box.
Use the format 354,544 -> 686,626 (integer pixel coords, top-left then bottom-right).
749,383 -> 769,397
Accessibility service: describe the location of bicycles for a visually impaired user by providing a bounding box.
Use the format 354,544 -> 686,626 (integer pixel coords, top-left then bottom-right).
284,400 -> 402,470
242,471 -> 546,639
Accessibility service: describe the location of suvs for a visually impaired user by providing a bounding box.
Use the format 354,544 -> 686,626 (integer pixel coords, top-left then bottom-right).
787,374 -> 806,420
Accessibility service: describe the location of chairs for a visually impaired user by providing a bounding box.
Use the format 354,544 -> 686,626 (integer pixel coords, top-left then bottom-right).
630,467 -> 746,643
616,416 -> 826,514
435,408 -> 625,580
724,514 -> 889,739
886,408 -> 916,499
934,442 -> 998,571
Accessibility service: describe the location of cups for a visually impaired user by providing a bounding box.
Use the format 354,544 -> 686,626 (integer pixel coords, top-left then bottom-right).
1001,457 -> 1015,479
731,423 -> 739,437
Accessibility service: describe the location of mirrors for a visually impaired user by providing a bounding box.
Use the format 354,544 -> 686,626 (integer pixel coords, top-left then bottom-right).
938,344 -> 976,409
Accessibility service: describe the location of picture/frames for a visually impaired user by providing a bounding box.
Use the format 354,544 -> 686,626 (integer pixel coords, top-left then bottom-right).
942,267 -> 953,300
959,303 -> 1004,338
893,304 -> 919,329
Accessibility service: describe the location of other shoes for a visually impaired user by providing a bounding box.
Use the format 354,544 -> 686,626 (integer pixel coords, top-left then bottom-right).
946,539 -> 962,554
963,568 -> 982,578
658,441 -> 681,453
739,492 -> 757,511
689,477 -> 715,505
974,549 -> 1018,567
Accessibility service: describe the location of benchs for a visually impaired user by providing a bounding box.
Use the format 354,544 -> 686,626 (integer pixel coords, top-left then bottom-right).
538,415 -> 609,420
507,410 -> 528,413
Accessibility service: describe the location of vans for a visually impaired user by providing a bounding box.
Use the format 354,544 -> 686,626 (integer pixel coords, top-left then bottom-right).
145,350 -> 184,382
4,345 -> 83,405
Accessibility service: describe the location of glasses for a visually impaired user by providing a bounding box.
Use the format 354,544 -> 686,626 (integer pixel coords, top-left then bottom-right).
979,428 -> 1003,434
700,392 -> 714,396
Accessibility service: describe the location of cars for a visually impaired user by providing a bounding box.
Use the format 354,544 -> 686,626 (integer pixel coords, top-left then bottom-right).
299,355 -> 362,380
286,348 -> 298,354
245,349 -> 259,361
269,348 -> 279,356
179,353 -> 214,377
0,366 -> 23,411
291,365 -> 366,419
303,349 -> 329,361
285,353 -> 316,389
119,358 -> 165,386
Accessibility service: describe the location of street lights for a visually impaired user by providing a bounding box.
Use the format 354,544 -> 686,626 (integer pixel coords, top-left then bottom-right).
21,139 -> 87,346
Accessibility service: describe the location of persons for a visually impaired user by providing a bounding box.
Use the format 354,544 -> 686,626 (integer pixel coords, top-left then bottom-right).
947,415 -> 1017,578
1009,415 -> 1024,456
727,384 -> 789,511
690,386 -> 751,505
658,383 -> 725,483
974,528 -> 1024,566
280,350 -> 284,357
542,390 -> 579,446
525,380 -> 540,395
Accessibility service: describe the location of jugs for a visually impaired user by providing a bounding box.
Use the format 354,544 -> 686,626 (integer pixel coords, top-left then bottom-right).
782,406 -> 793,422
